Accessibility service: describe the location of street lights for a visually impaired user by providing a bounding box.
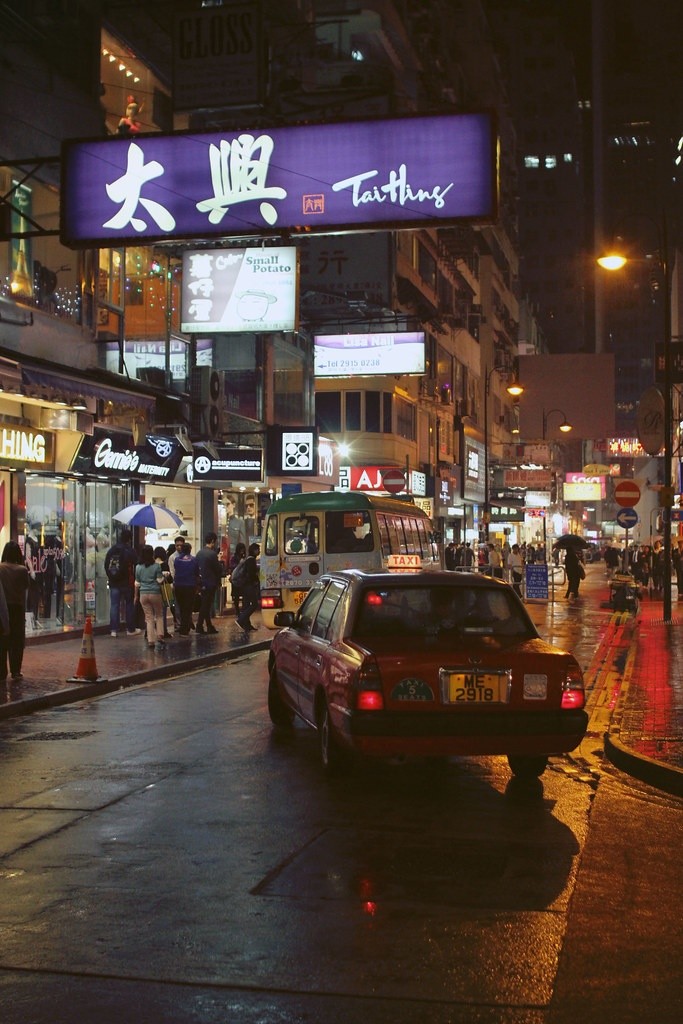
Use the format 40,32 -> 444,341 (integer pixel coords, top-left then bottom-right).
484,365 -> 524,544
543,410 -> 573,556
597,212 -> 672,622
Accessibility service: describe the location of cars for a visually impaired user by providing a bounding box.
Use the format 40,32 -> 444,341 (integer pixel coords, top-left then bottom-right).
561,548 -> 592,563
267,568 -> 588,778
587,542 -> 601,561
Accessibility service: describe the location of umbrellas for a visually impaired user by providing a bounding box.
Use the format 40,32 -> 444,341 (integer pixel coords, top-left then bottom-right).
640,535 -> 664,545
111,502 -> 184,530
552,534 -> 591,549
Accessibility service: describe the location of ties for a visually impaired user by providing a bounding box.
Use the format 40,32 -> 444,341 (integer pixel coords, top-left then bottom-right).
633,551 -> 638,562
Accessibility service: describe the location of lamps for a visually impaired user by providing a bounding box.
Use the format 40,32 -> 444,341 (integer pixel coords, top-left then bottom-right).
151,423 -> 194,453
191,440 -> 221,461
72,398 -> 88,410
30,386 -> 48,401
14,386 -> 27,397
53,395 -> 68,406
344,290 -> 369,316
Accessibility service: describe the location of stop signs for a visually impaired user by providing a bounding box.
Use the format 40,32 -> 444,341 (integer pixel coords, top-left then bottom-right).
614,481 -> 641,508
382,470 -> 405,493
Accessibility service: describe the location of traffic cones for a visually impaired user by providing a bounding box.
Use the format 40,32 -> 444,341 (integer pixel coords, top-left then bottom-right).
66,619 -> 108,684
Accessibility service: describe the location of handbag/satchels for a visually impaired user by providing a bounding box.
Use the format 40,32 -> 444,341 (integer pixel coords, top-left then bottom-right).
577,564 -> 584,581
158,579 -> 174,607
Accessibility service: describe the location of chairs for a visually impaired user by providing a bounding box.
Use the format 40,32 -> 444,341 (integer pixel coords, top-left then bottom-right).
360,533 -> 373,552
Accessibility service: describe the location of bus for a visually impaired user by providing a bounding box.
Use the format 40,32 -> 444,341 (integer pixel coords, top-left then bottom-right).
259,492 -> 441,629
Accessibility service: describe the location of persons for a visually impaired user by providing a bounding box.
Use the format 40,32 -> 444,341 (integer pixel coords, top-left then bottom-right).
478,541 -> 544,598
25,521 -> 42,617
133,532 -> 260,647
445,542 -> 475,572
223,493 -> 271,554
604,541 -> 683,594
0,540 -> 31,680
552,548 -> 580,598
116,103 -> 142,134
104,529 -> 142,637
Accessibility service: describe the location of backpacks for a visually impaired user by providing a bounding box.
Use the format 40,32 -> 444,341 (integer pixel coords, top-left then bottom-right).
229,556 -> 254,586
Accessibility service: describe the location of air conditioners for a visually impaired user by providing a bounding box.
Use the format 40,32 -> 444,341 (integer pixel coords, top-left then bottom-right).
441,388 -> 451,405
461,399 -> 471,417
499,415 -> 506,423
427,379 -> 438,396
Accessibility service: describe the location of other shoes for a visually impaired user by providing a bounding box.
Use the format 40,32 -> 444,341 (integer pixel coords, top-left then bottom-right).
11,673 -> 23,678
247,625 -> 258,631
206,626 -> 218,633
234,621 -> 245,632
163,631 -> 172,638
196,626 -> 206,633
179,633 -> 190,638
110,631 -> 117,637
126,628 -> 141,635
